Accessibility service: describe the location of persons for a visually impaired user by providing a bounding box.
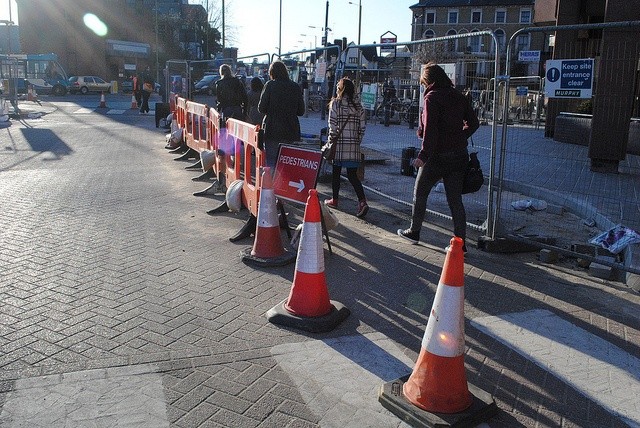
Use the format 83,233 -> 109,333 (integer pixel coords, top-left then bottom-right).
132,74 -> 141,109
397,64 -> 482,256
158,69 -> 167,103
257,60 -> 305,187
139,65 -> 155,113
321,77 -> 370,218
215,63 -> 247,128
527,98 -> 536,119
247,77 -> 264,155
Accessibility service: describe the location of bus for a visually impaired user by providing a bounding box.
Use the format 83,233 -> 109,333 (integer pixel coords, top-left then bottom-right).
1,54 -> 71,96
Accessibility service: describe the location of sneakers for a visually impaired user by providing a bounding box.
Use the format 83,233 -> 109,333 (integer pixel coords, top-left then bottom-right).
397,228 -> 420,243
146,111 -> 151,115
324,198 -> 338,209
139,112 -> 145,115
357,199 -> 369,217
444,243 -> 467,255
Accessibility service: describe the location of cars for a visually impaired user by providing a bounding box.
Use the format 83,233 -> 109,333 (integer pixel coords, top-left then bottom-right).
122,82 -> 161,93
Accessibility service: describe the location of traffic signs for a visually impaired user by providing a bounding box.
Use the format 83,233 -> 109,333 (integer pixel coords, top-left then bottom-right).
271,143 -> 324,206
545,57 -> 594,99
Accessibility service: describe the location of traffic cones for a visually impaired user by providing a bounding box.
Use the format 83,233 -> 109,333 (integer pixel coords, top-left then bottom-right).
241,167 -> 298,267
129,90 -> 140,110
98,90 -> 108,109
267,189 -> 349,334
377,236 -> 498,428
27,84 -> 34,101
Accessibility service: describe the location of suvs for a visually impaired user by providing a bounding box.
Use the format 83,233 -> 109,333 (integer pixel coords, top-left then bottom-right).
67,76 -> 111,94
192,75 -> 221,96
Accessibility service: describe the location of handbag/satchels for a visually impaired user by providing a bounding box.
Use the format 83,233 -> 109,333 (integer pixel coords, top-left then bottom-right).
141,72 -> 153,92
321,114 -> 351,161
443,131 -> 484,194
